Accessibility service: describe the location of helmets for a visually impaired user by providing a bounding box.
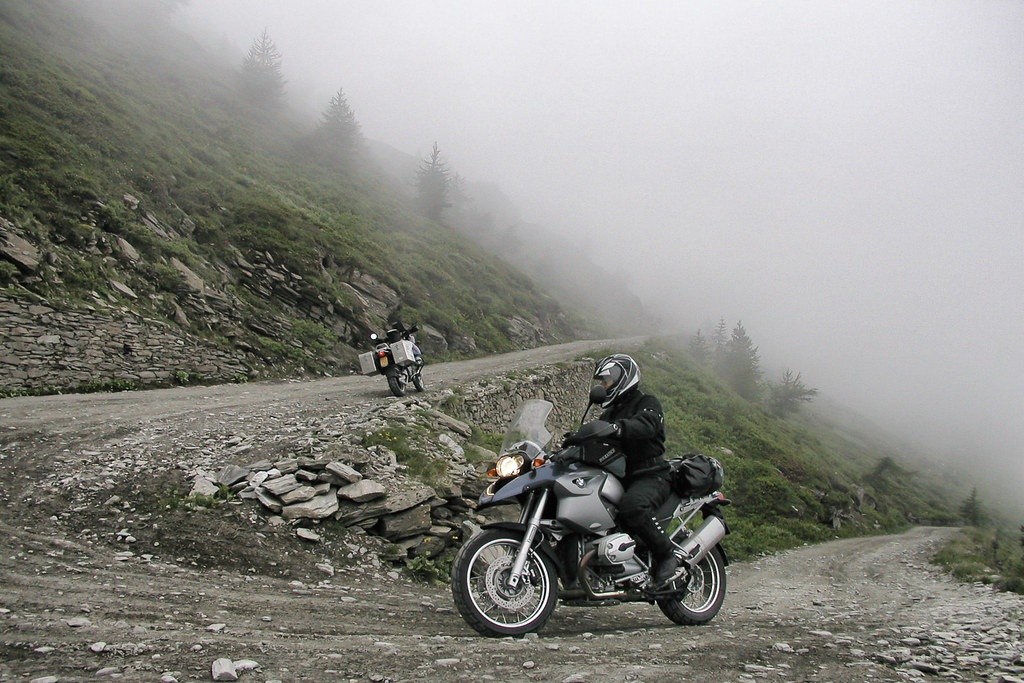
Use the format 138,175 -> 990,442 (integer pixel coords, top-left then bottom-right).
593,354 -> 641,408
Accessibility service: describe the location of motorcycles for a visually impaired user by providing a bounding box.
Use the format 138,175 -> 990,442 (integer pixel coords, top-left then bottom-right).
360,324 -> 427,396
450,354 -> 733,637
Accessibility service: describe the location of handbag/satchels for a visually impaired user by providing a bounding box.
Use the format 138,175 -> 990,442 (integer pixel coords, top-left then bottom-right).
672,452 -> 724,498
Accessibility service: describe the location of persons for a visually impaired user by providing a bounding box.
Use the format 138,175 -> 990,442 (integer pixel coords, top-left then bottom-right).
564,353 -> 678,581
385,314 -> 422,361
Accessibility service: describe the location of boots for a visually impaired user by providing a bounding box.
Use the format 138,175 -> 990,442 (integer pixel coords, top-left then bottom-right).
637,513 -> 679,591
